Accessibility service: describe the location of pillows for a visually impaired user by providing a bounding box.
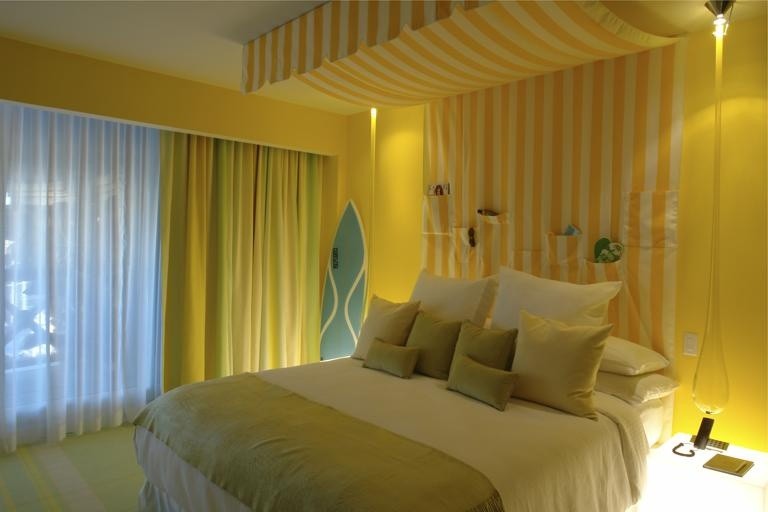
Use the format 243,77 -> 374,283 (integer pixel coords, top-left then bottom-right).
360,337 -> 421,380
598,331 -> 670,377
445,351 -> 520,410
597,374 -> 681,407
509,309 -> 616,422
352,292 -> 421,365
406,269 -> 492,325
450,317 -> 516,369
407,308 -> 460,381
486,264 -> 622,329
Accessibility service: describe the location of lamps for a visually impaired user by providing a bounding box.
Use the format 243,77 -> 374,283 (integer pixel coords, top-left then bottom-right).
706,1 -> 736,37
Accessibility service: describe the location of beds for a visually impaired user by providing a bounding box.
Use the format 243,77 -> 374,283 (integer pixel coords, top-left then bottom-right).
131,356 -> 672,512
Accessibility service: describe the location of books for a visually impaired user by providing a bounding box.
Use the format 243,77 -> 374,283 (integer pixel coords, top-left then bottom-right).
702,453 -> 756,478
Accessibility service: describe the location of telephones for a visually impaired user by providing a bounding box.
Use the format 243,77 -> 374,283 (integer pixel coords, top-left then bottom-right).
673,418 -> 715,457
